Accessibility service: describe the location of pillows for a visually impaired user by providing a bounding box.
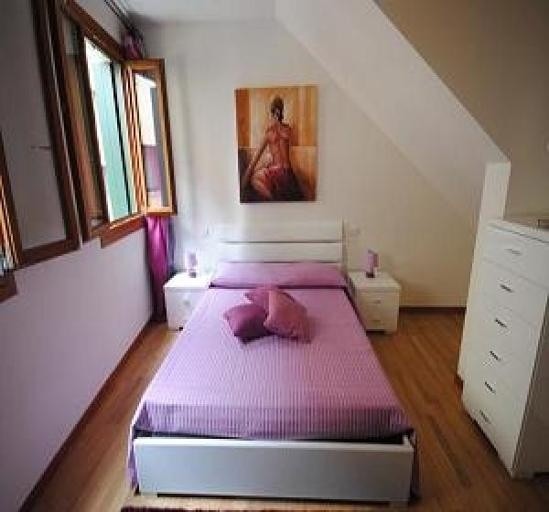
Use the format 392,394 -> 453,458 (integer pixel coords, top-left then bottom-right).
212,262 -> 348,287
223,285 -> 310,344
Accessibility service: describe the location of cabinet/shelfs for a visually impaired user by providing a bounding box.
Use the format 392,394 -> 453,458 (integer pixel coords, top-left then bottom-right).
461,216 -> 549,480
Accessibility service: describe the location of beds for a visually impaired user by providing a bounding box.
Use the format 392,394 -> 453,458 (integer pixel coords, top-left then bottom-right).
128,219 -> 422,507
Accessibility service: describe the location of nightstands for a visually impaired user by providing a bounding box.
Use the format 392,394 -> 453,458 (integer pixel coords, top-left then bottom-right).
348,271 -> 402,334
163,272 -> 213,330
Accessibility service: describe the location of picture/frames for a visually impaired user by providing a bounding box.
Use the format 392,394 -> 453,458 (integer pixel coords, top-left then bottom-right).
233,81 -> 321,204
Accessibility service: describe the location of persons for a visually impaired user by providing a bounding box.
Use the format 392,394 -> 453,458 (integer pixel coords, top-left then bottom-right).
241,95 -> 312,200
